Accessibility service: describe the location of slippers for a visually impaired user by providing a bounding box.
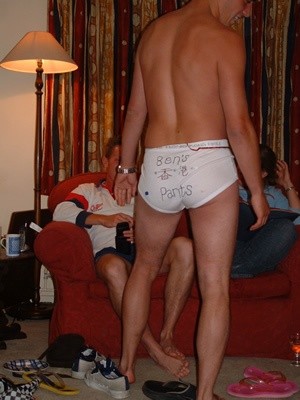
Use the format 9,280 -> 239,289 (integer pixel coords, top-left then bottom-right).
142,380 -> 197,400
22,370 -> 80,396
243,366 -> 300,393
226,377 -> 294,398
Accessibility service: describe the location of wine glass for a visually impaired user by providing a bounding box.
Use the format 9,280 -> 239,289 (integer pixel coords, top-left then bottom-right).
288,329 -> 300,368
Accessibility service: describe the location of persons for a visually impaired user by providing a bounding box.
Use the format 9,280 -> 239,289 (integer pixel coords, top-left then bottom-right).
114,0 -> 269,400
231,143 -> 300,277
53,141 -> 194,378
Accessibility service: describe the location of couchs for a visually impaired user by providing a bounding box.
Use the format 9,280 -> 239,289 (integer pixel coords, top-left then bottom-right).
33,172 -> 300,361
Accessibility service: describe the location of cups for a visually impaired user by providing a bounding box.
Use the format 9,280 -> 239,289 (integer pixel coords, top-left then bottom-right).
1,234 -> 21,256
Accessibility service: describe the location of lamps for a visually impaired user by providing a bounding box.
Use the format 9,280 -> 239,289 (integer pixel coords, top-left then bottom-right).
0,31 -> 78,320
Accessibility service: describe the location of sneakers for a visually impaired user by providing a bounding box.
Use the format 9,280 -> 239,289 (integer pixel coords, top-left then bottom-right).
84,366 -> 130,399
71,347 -> 118,379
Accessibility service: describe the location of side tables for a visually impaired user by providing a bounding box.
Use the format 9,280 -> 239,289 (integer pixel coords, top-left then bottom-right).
0,248 -> 37,320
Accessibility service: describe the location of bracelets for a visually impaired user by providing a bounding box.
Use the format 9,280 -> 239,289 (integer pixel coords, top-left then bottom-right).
285,187 -> 294,191
117,166 -> 135,174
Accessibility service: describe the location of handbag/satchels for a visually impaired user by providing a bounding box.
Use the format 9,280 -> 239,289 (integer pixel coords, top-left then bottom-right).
38,334 -> 85,377
